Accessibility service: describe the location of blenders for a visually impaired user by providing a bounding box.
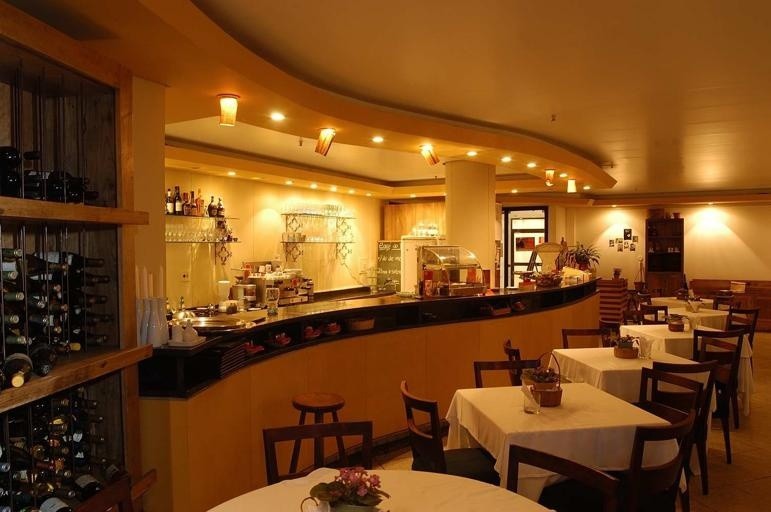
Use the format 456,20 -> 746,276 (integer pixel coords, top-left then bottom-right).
217,280 -> 231,313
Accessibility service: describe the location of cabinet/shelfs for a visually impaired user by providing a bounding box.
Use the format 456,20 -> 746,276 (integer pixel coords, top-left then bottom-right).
281,212 -> 359,244
164,215 -> 241,244
645,216 -> 686,272
240,260 -> 313,306
1,0 -> 160,510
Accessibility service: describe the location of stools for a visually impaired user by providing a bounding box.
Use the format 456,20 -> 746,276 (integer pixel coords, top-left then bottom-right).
289,391 -> 349,476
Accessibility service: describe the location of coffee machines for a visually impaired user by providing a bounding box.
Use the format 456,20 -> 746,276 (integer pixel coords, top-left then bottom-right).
233,283 -> 258,310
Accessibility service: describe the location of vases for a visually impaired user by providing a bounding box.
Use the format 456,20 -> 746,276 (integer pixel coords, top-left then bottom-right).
529,387 -> 563,408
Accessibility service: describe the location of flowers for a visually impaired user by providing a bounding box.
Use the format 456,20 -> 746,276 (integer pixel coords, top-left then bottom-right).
520,364 -> 561,388
324,460 -> 388,504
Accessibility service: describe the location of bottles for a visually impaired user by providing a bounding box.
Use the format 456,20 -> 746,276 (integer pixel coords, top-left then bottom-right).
553,275 -> 561,286
667,245 -> 680,253
167,185 -> 225,217
418,280 -> 424,296
649,223 -> 657,236
276,266 -> 281,275
184,320 -> 198,342
139,296 -> 170,348
0,384 -> 131,512
0,246 -> 111,388
566,277 -> 573,285
0,145 -> 100,204
243,264 -> 252,284
437,285 -> 441,296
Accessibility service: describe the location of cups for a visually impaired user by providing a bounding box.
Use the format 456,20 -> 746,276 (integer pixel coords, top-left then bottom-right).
172,325 -> 184,345
688,316 -> 702,331
265,287 -> 280,314
258,264 -> 272,273
282,232 -> 306,242
425,279 -> 433,296
639,339 -> 653,360
524,390 -> 542,414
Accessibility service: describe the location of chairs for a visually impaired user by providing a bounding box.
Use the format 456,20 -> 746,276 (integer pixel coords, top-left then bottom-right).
261,421 -> 374,484
638,366 -> 705,428
507,440 -> 622,511
559,280 -> 771,376
502,337 -> 527,386
473,358 -> 542,389
689,328 -> 748,468
608,407 -> 699,512
651,360 -> 718,428
399,378 -> 496,483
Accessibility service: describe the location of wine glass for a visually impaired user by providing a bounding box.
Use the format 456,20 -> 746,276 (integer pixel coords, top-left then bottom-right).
165,224 -> 214,243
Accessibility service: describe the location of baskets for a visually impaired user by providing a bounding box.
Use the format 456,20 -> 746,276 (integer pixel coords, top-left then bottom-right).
523,352 -> 562,407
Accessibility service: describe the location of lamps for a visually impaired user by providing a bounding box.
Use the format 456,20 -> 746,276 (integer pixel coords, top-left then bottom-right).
410,222 -> 440,236
566,177 -> 577,193
314,128 -> 335,157
419,141 -> 440,169
217,94 -> 240,127
544,168 -> 556,189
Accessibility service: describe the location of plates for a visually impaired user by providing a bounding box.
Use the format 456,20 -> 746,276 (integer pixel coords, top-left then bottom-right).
507,287 -> 519,289
